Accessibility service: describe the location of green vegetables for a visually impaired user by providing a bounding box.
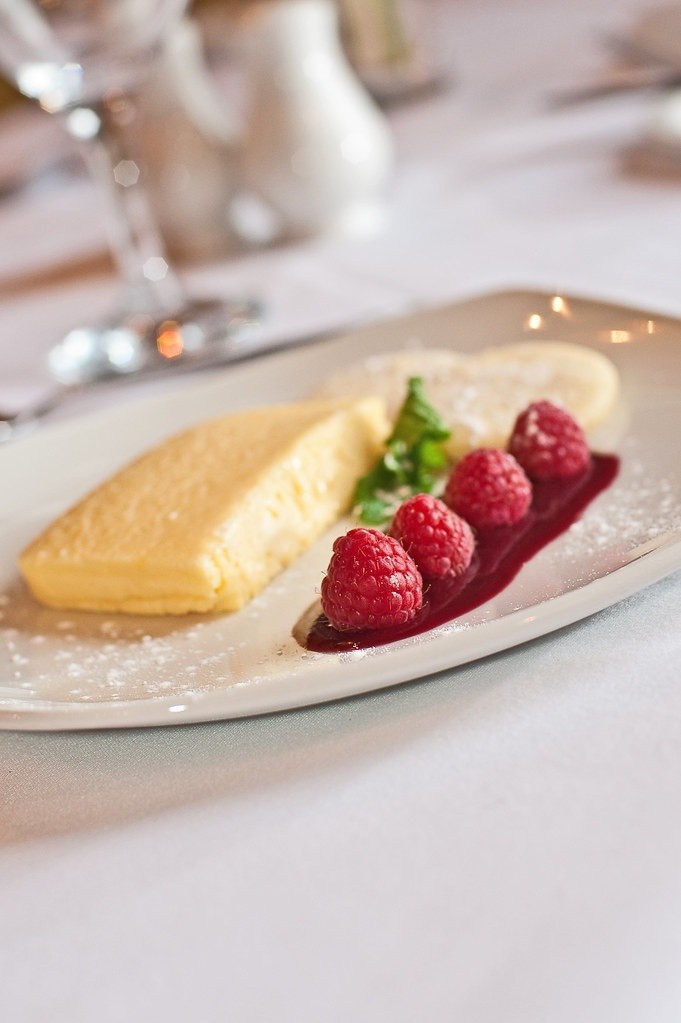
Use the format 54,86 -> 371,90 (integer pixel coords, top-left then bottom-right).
355,377 -> 451,527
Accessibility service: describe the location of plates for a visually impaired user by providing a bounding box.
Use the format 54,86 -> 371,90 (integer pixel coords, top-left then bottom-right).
0,290 -> 681,732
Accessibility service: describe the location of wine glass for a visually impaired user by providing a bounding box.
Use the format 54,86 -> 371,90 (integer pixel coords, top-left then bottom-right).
0,0 -> 274,381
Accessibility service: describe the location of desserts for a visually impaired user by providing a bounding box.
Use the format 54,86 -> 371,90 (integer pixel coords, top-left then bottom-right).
16,397 -> 389,619
